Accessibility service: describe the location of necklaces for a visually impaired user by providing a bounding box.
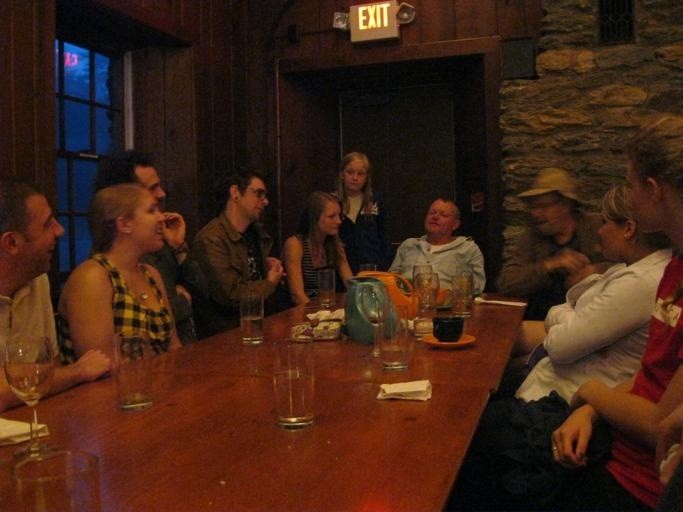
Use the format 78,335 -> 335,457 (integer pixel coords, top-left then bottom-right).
121,272 -> 151,304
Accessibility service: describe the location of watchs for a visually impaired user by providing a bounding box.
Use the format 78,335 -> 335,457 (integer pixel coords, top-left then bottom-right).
172,241 -> 190,256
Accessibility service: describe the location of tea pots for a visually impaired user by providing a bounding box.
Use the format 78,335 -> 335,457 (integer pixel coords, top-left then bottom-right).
344,277 -> 398,346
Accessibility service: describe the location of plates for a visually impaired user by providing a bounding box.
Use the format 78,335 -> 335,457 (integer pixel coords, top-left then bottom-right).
423,335 -> 475,349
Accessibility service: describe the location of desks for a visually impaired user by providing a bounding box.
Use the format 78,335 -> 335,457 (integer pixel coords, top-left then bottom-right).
1,291 -> 527,512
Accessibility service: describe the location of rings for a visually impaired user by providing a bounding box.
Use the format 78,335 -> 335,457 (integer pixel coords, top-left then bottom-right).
552,447 -> 557,451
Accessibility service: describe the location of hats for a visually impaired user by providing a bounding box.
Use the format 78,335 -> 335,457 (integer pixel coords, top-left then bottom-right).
516,167 -> 591,209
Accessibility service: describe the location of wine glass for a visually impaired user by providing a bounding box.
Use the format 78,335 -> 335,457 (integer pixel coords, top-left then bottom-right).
4,336 -> 62,462
358,263 -> 383,271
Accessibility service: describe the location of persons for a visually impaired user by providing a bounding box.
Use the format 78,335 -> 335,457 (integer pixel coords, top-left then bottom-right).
388,198 -> 486,294
330,151 -> 395,293
539,112 -> 683,512
0,177 -> 112,414
57,184 -> 182,369
277,191 -> 355,312
494,166 -> 618,320
657,406 -> 683,510
499,185 -> 682,405
191,170 -> 299,342
96,151 -> 209,346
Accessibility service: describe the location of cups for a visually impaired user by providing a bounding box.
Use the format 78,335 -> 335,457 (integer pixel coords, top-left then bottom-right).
113,331 -> 154,412
272,338 -> 315,427
11,448 -> 100,512
411,264 -> 475,319
317,268 -> 336,307
431,317 -> 464,342
378,304 -> 409,372
239,292 -> 265,345
